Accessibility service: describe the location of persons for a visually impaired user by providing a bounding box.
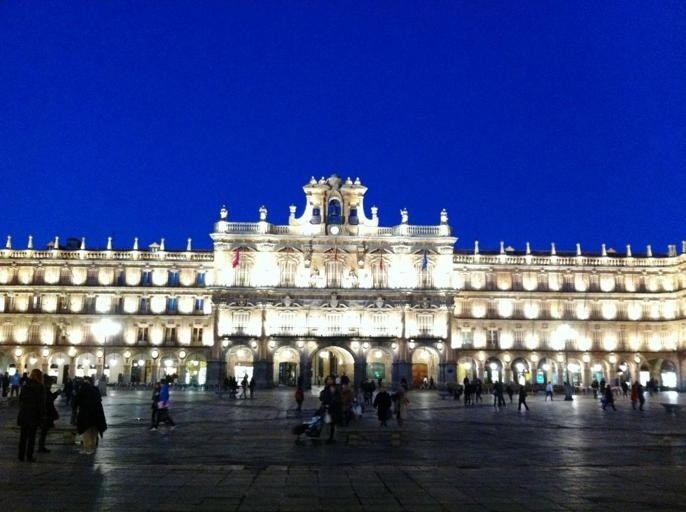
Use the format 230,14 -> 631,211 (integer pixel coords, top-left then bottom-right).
294,370 -> 408,444
415,375 -> 659,412
0,369 -> 256,462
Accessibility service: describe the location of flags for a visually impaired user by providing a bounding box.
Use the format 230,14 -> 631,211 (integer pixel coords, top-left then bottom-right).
421,247 -> 428,271
232,248 -> 240,268
